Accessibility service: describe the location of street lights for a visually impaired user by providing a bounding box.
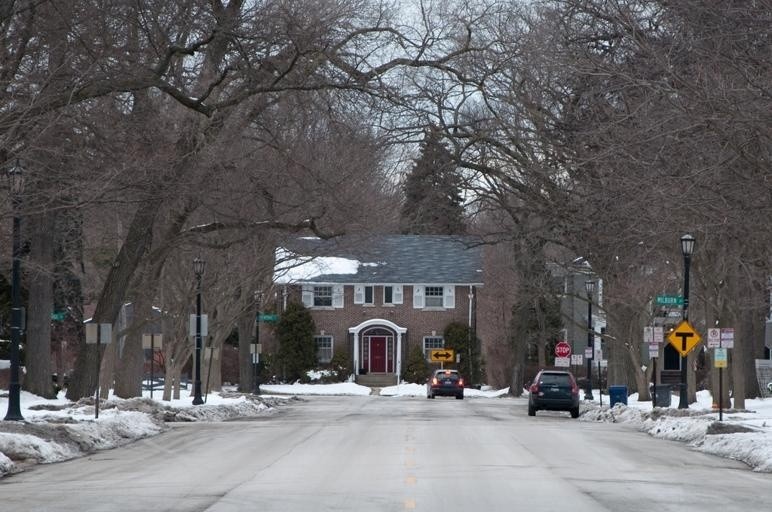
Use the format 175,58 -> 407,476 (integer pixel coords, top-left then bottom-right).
676,232 -> 697,409
466,293 -> 475,385
249,286 -> 266,396
0,156 -> 30,421
191,248 -> 209,406
583,273 -> 596,399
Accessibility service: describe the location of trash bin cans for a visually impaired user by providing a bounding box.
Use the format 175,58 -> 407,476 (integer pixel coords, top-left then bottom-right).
649,384 -> 672,407
609,385 -> 628,408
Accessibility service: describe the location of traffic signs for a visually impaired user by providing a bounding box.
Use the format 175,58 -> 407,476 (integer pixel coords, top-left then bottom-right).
256,315 -> 278,321
656,295 -> 684,307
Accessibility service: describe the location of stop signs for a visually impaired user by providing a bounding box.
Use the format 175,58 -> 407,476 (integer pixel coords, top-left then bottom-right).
555,341 -> 571,359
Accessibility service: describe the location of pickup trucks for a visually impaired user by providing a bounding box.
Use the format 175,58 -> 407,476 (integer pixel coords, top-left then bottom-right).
51,369 -> 73,389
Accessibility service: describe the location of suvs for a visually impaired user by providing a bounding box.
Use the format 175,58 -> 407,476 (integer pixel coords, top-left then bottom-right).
527,368 -> 581,419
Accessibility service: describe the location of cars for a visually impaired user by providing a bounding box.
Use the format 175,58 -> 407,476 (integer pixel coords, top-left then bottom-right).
425,368 -> 465,400
